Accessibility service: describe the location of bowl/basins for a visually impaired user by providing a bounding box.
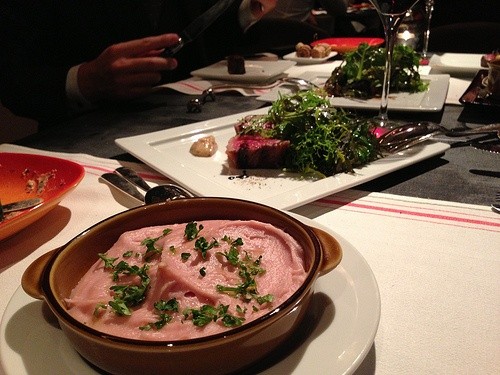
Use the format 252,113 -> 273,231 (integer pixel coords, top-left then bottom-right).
20,195 -> 343,375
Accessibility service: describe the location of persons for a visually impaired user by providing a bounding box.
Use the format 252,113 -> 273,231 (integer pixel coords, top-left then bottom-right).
0,0 -> 347,132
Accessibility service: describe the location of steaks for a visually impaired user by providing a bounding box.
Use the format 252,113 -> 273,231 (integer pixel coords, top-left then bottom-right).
226,115 -> 290,169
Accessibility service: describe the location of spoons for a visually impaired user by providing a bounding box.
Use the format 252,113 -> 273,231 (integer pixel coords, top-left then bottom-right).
101,166 -> 193,205
374,120 -> 500,160
212,76 -> 319,92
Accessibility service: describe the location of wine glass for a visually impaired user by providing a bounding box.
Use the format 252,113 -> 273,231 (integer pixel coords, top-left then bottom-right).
410,0 -> 442,58
368,0 -> 418,128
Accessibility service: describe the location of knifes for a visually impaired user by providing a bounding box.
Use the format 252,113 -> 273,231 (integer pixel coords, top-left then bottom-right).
0,198 -> 43,214
157,0 -> 236,59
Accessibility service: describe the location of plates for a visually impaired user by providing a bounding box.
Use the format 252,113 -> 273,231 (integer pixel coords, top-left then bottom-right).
190,57 -> 298,83
283,49 -> 338,65
113,98 -> 450,211
0,209 -> 383,375
429,52 -> 490,71
0,151 -> 86,242
310,37 -> 385,55
254,70 -> 450,112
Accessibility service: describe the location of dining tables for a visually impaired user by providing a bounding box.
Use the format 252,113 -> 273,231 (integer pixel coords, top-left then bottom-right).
0,52 -> 500,375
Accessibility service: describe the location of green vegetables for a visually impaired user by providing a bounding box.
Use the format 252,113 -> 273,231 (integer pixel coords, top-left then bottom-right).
239,84 -> 398,182
91,220 -> 273,331
323,42 -> 429,99
